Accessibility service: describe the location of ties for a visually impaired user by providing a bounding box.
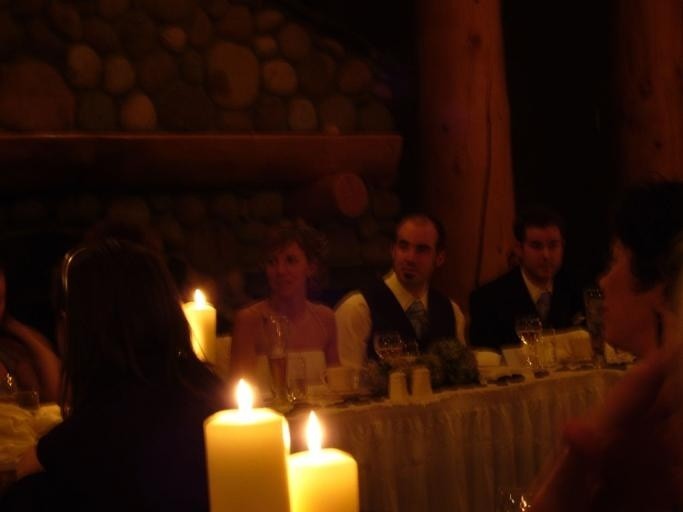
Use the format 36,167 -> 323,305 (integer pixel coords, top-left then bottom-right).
411,300 -> 428,336
534,291 -> 551,326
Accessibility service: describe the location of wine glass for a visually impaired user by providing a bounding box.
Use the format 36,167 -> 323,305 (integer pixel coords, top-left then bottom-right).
514,319 -> 543,368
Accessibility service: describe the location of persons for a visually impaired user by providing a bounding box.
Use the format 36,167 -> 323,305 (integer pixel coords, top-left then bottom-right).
523,182 -> 682,510
234,212 -> 583,407
1,182 -> 226,510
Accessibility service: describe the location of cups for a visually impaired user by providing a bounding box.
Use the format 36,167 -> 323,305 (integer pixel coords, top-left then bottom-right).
389,372 -> 409,405
372,327 -> 403,366
320,366 -> 356,391
412,368 -> 434,400
502,348 -> 523,371
263,314 -> 293,407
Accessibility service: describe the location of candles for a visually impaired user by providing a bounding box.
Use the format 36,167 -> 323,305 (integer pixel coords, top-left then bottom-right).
182,289 -> 216,366
203,377 -> 292,512
289,410 -> 360,512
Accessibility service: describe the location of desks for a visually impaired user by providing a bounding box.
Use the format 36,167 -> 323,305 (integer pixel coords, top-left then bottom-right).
0,364 -> 634,512
